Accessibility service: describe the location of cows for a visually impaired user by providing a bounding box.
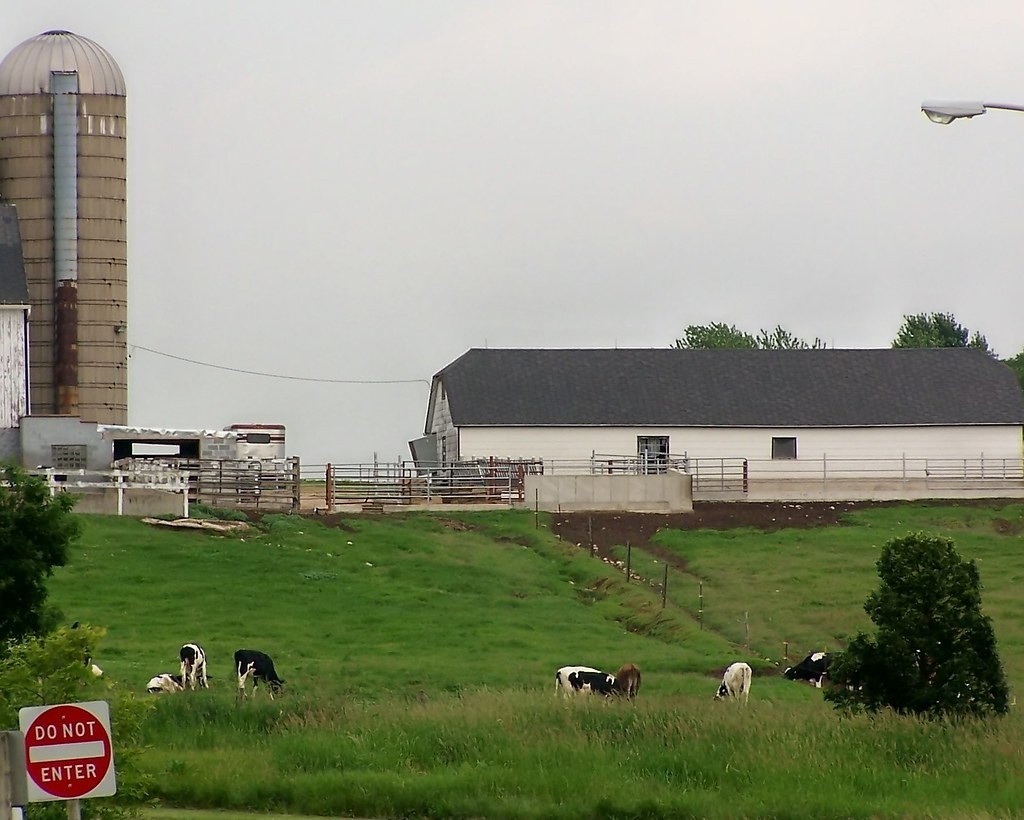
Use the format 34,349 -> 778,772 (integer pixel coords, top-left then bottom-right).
554,665 -> 601,693
233,648 -> 288,699
712,661 -> 753,703
145,672 -> 212,694
568,670 -> 635,700
616,663 -> 642,701
178,642 -> 211,692
784,652 -> 833,689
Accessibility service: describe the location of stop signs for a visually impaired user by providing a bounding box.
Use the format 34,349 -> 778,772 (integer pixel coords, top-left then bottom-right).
18,699 -> 119,804
